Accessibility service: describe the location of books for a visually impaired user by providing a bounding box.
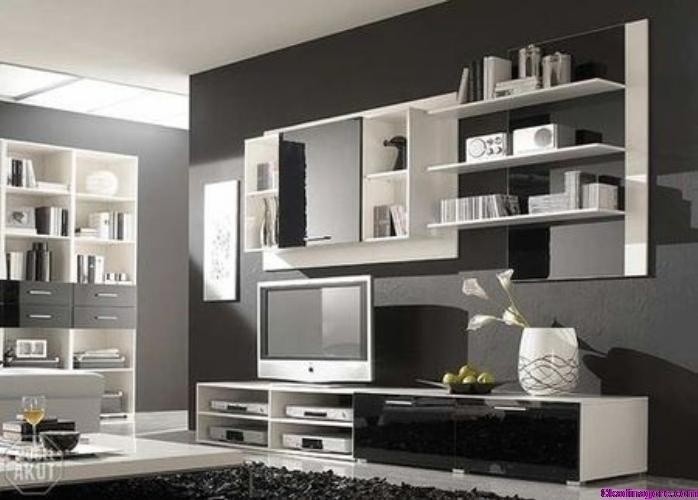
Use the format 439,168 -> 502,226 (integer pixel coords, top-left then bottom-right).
2,150 -> 137,461
368,31 -> 627,245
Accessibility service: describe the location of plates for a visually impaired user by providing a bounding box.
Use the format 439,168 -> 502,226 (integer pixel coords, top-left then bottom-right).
416,377 -> 518,395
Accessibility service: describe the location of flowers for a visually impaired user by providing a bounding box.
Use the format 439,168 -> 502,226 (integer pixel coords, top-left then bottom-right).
461,268 -> 533,331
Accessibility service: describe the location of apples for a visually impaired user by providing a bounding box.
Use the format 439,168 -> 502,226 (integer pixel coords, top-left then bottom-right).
442,365 -> 496,384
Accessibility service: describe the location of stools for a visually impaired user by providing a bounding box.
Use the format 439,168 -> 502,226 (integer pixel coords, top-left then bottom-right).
0,367 -> 105,441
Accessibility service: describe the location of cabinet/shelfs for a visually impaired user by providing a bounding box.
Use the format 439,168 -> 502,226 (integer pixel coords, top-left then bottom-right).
0,139 -> 139,427
195,381 -> 650,487
244,76 -> 625,254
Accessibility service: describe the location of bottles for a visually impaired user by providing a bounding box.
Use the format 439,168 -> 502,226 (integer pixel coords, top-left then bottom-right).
518,45 -> 571,88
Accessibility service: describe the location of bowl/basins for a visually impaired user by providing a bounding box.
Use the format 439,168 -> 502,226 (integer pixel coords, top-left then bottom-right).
38,431 -> 80,452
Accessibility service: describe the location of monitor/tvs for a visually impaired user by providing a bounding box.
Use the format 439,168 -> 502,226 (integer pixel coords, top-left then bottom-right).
256,275 -> 376,386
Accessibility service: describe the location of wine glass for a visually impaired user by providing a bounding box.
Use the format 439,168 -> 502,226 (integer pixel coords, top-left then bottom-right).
22,396 -> 44,451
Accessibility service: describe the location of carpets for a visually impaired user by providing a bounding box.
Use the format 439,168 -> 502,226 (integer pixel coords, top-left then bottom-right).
0,460 -> 539,500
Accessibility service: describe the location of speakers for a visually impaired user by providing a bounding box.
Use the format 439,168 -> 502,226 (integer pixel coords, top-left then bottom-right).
511,123 -> 575,158
465,132 -> 508,161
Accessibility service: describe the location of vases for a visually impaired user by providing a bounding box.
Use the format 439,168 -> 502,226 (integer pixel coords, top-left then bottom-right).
517,327 -> 581,397
